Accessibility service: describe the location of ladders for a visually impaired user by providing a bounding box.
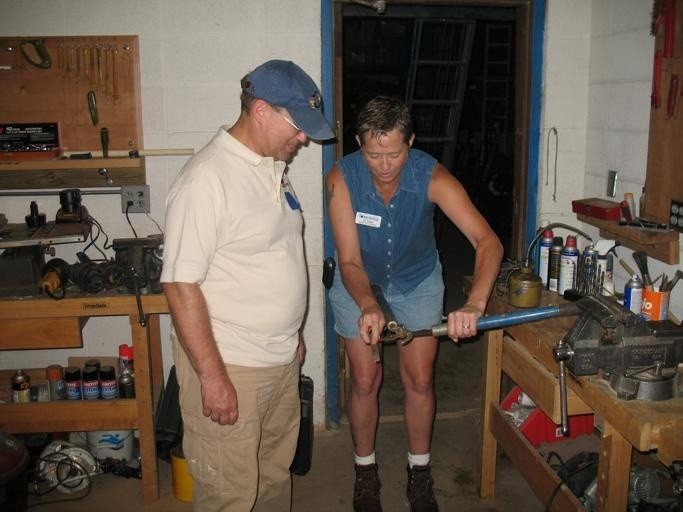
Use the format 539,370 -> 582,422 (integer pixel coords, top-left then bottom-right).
404,17 -> 477,172
480,22 -> 511,163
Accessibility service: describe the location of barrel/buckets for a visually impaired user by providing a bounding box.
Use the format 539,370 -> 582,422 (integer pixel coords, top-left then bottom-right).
86,430 -> 134,463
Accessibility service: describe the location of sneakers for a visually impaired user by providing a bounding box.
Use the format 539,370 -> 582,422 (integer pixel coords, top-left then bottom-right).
353,463 -> 383,512
406,463 -> 440,512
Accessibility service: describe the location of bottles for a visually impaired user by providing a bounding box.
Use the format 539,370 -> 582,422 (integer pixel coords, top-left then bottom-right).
36,344 -> 137,402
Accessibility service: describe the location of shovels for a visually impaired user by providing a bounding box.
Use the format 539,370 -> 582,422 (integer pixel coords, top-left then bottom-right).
632,250 -> 651,285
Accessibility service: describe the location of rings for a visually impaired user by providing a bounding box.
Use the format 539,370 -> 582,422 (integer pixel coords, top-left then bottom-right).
462,325 -> 470,330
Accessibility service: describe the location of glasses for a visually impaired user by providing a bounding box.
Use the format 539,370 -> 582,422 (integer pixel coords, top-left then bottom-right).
268,104 -> 301,135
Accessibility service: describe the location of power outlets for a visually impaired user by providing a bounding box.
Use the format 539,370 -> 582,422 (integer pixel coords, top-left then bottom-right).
122,186 -> 149,214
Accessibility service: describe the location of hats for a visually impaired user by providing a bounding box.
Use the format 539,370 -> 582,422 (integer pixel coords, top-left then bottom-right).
241,60 -> 335,141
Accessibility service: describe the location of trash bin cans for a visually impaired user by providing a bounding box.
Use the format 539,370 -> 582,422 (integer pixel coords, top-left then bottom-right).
168,440 -> 192,501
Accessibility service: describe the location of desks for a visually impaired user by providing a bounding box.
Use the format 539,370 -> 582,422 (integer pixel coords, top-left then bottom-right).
462,275 -> 683,511
0,280 -> 173,504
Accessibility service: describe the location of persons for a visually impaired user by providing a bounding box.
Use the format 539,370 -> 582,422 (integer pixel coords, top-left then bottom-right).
327,96 -> 504,512
160,59 -> 336,512
